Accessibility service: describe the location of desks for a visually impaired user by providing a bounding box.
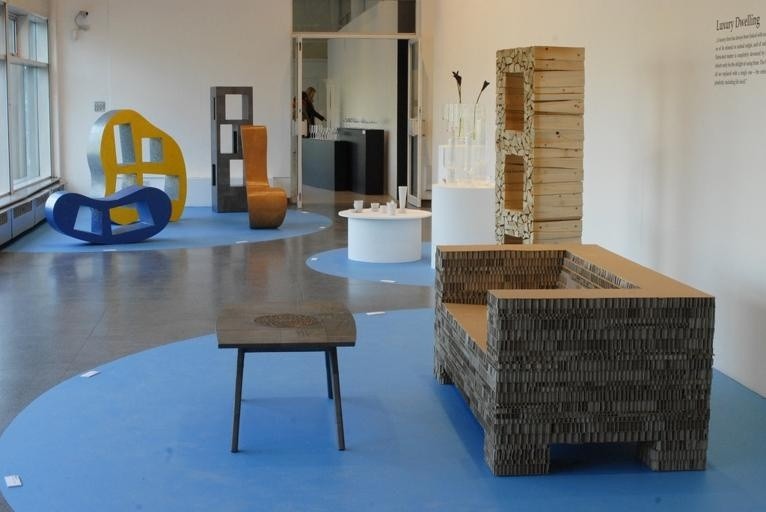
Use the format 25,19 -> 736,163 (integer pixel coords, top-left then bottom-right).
338,202 -> 429,264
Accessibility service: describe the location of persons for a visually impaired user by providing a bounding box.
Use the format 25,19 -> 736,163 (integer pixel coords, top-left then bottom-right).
294,91 -> 311,137
306,87 -> 327,125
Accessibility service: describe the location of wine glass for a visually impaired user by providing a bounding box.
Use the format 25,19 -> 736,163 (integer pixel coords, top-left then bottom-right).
309,116 -> 377,140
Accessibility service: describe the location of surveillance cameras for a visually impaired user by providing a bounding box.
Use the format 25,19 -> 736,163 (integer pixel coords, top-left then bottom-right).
79,9 -> 88,18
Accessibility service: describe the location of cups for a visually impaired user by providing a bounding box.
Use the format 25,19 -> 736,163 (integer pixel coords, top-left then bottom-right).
354,200 -> 363,212
370,201 -> 397,215
398,186 -> 407,214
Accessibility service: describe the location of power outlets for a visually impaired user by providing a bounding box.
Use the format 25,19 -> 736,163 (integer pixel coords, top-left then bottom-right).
95,102 -> 105,112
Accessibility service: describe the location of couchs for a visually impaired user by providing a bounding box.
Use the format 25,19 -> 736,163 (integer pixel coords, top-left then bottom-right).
435,243 -> 717,473
